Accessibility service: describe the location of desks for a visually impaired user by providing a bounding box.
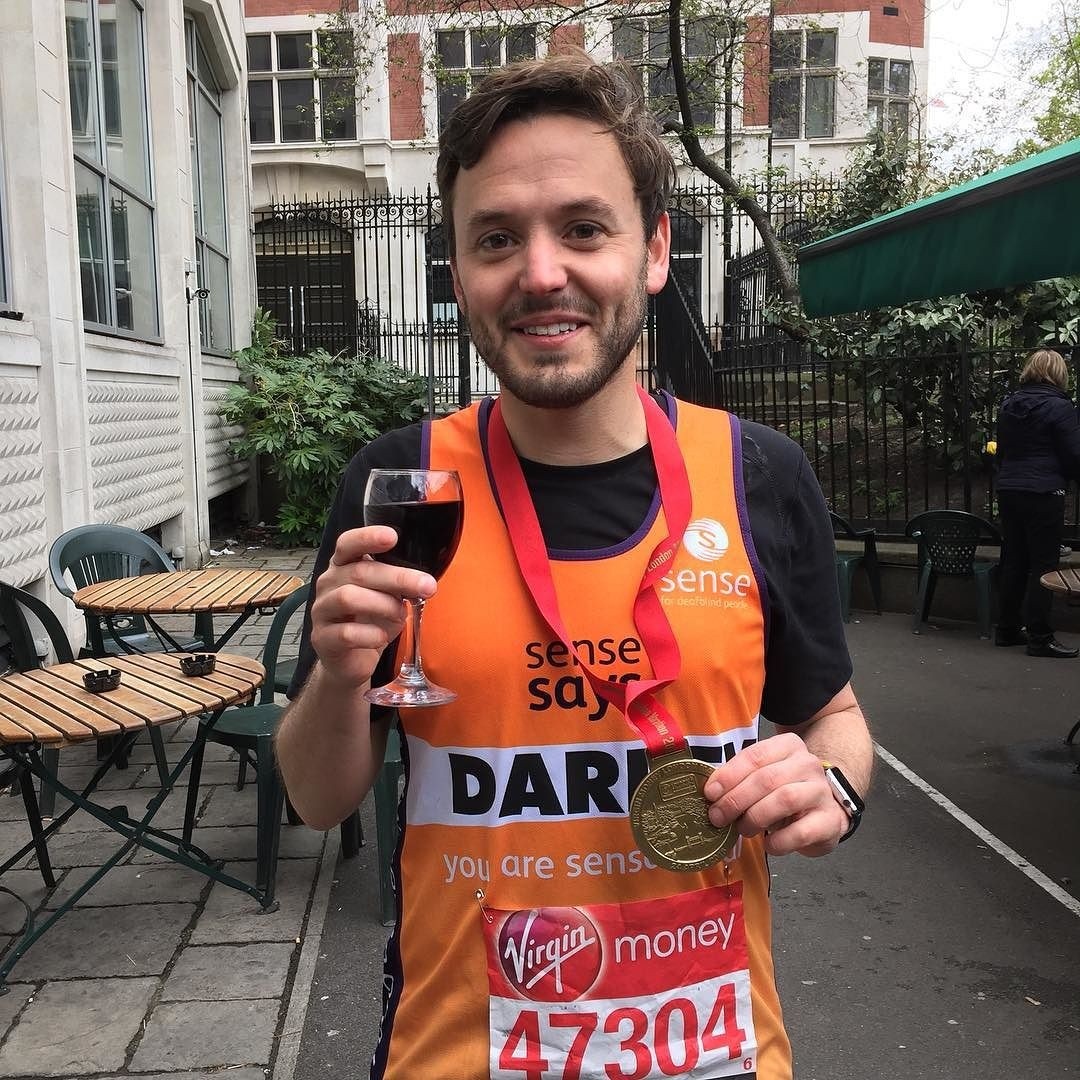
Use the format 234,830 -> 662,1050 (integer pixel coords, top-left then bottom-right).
1040,568 -> 1080,775
73,569 -> 305,771
0,653 -> 266,887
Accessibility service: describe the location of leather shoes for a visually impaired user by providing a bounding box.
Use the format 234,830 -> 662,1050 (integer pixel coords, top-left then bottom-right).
1028,635 -> 1079,658
996,630 -> 1030,645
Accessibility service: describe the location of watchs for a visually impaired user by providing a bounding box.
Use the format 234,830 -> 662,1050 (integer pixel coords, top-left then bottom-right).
821,758 -> 865,843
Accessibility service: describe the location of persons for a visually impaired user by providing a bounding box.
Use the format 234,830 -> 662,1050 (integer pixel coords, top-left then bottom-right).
279,58 -> 873,1080
994,351 -> 1080,657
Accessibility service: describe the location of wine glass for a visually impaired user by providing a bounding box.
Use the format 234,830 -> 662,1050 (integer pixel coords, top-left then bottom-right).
363,469 -> 465,707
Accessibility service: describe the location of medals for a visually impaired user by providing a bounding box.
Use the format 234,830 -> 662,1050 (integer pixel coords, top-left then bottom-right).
629,759 -> 741,872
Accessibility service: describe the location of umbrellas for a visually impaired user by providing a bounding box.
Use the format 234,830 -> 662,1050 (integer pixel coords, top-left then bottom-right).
797,135 -> 1080,317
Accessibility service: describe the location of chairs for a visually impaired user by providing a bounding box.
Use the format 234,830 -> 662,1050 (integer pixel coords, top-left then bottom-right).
829,510 -> 882,623
905,510 -> 1001,640
0,580 -> 171,820
179,584 -> 364,886
275,656 -> 366,848
48,523 -> 177,768
